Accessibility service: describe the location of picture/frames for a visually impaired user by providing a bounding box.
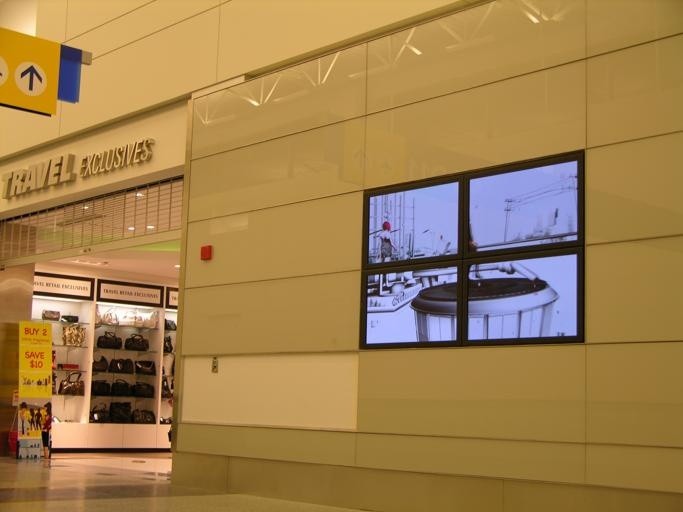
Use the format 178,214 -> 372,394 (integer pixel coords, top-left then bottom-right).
362,171 -> 463,270
463,149 -> 585,259
359,260 -> 462,349
462,247 -> 585,346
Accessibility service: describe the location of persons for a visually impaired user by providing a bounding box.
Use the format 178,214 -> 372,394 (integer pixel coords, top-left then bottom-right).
19,401 -> 52,459
379,221 -> 397,289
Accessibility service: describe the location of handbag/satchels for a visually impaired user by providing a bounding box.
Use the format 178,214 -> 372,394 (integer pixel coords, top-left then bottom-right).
42,306 -> 176,425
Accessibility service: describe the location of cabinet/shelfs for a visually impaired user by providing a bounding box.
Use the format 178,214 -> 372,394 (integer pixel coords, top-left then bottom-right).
31,296 -> 178,448
